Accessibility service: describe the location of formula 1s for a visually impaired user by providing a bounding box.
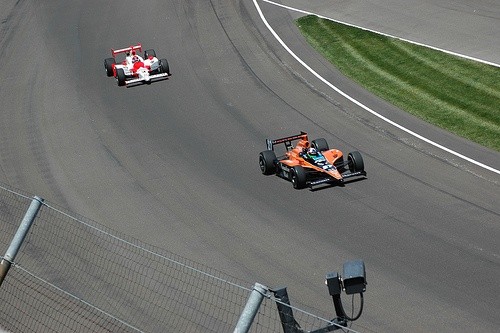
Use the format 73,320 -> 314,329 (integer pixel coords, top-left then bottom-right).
258,131 -> 369,191
104,42 -> 171,90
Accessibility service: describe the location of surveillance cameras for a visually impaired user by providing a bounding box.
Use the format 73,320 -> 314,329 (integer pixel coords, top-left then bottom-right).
342,259 -> 367,295
